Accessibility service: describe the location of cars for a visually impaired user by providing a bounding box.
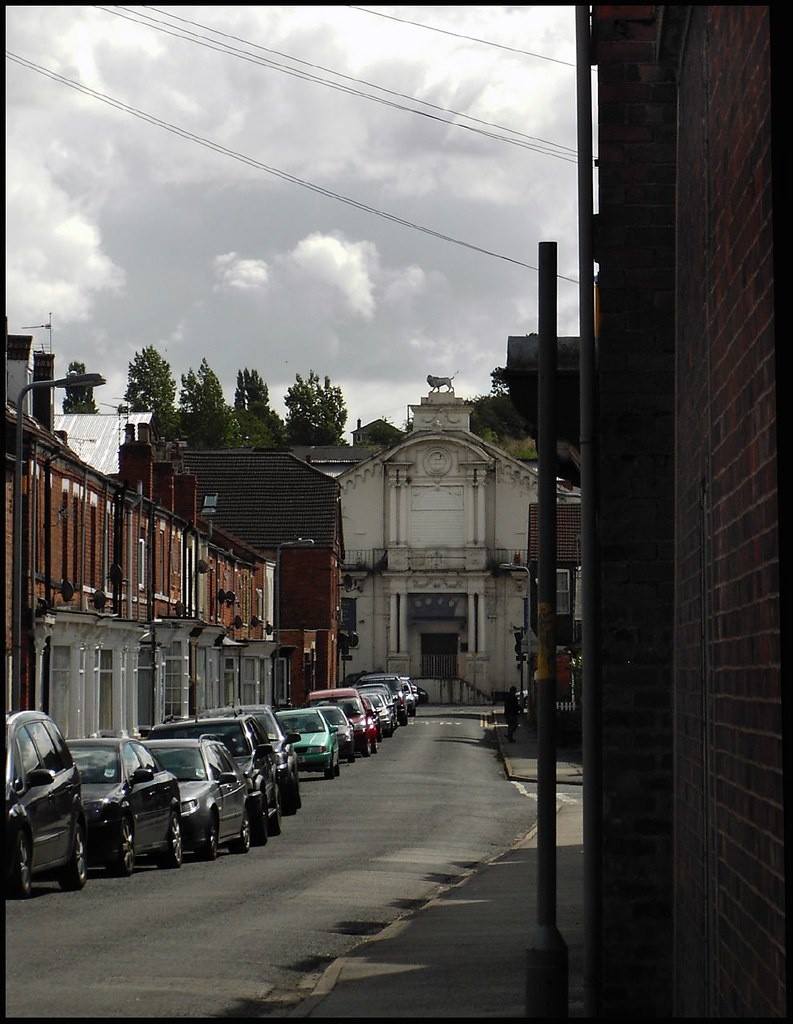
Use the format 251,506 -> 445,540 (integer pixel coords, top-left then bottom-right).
134,734 -> 251,860
275,709 -> 340,779
360,693 -> 395,738
399,677 -> 419,717
303,706 -> 355,762
317,697 -> 382,753
65,737 -> 183,877
408,680 -> 428,703
357,684 -> 399,729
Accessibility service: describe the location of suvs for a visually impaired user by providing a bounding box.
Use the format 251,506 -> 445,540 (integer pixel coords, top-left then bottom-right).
197,701 -> 302,816
306,688 -> 374,756
145,708 -> 282,846
357,673 -> 407,726
6,710 -> 87,898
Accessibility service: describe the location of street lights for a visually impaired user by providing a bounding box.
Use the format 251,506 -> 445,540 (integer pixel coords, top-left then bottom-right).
499,563 -> 531,721
276,538 -> 315,711
11,373 -> 106,713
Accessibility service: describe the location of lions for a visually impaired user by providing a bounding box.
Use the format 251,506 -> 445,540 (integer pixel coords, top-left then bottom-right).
427,373 -> 458,393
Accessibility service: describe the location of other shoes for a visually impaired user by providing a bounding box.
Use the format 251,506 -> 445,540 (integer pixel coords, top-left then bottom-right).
504,734 -> 516,743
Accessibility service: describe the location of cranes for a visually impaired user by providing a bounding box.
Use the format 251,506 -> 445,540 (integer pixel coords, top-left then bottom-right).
21,312 -> 52,354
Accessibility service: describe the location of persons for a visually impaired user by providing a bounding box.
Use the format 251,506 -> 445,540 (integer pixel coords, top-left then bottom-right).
505,686 -> 520,742
